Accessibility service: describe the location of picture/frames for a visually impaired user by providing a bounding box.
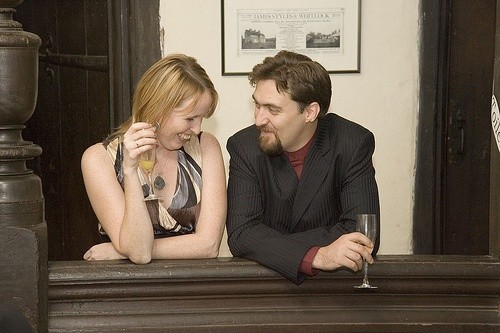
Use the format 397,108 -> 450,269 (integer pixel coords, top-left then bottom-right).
220,0 -> 362,76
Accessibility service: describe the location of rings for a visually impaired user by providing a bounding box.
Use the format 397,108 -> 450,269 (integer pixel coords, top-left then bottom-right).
136,141 -> 138,147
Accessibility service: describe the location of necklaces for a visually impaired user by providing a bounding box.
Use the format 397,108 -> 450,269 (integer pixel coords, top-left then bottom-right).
153,150 -> 170,190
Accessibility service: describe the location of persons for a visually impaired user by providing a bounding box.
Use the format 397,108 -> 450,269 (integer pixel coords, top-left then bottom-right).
226,50 -> 380,285
81,53 -> 227,265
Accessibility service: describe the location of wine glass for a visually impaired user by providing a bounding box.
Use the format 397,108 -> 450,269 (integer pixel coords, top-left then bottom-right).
135,127 -> 164,201
353,214 -> 378,288
